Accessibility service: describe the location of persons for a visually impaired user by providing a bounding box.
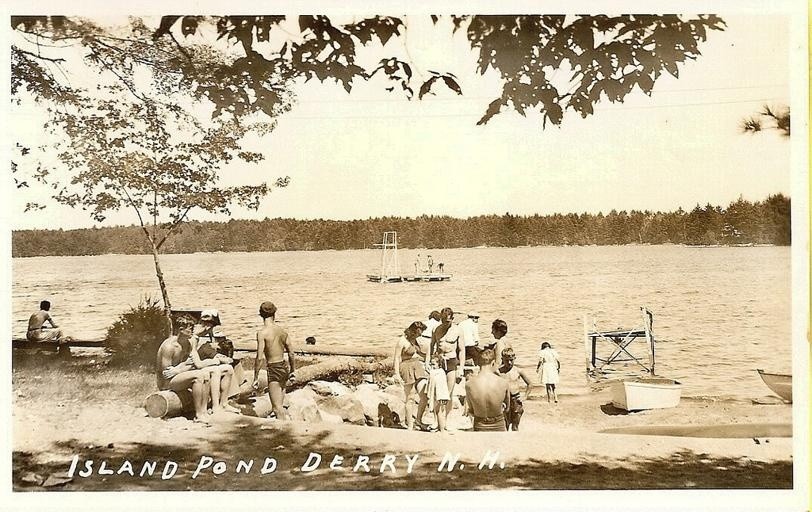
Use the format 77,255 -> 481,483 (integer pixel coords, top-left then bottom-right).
306,337 -> 315,344
427,255 -> 433,274
536,342 -> 562,404
393,321 -> 428,431
420,308 -> 532,431
438,262 -> 445,273
414,253 -> 423,276
27,301 -> 63,344
251,301 -> 296,422
154,311 -> 247,426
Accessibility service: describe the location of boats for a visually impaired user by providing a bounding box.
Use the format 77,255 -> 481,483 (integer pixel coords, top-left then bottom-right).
611,375 -> 682,412
757,369 -> 792,403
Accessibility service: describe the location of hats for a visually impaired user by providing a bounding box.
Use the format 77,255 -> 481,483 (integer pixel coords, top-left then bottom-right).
200,307 -> 222,326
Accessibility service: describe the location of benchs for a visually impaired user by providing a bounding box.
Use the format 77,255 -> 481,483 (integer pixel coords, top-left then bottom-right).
14,336 -> 105,358
233,338 -> 382,362
